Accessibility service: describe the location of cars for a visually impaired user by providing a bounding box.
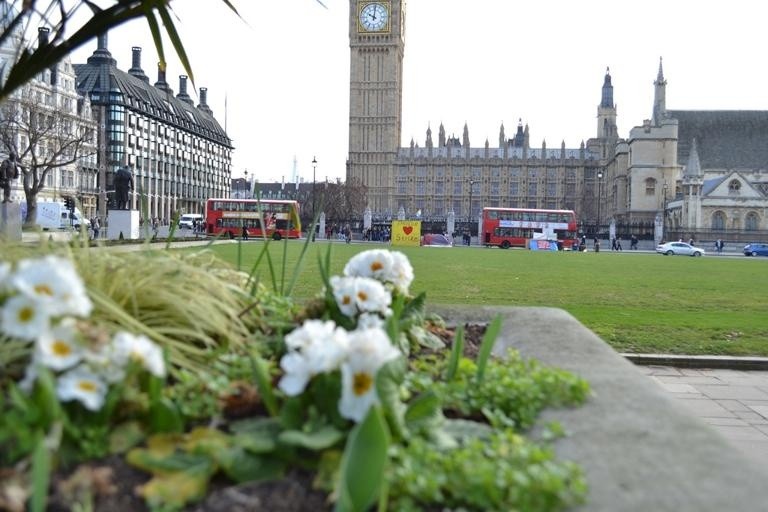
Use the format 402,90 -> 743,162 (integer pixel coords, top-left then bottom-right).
654,241 -> 706,257
744,243 -> 767,256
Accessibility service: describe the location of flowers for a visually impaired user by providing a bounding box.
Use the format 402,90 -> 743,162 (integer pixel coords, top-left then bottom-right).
0,256 -> 166,412
278,247 -> 414,424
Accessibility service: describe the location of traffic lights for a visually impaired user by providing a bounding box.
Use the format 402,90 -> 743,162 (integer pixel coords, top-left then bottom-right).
68,198 -> 72,210
63,197 -> 68,207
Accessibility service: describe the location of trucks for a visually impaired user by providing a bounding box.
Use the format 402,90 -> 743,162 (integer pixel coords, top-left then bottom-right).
179,213 -> 203,229
21,202 -> 90,230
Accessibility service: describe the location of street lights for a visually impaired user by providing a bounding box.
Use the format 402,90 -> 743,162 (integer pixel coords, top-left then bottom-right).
596,170 -> 603,235
244,169 -> 248,198
660,179 -> 667,240
312,154 -> 318,220
467,177 -> 475,222
235,180 -> 240,199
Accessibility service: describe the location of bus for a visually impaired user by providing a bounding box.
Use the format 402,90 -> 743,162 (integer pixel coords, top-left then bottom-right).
206,197 -> 301,241
480,205 -> 578,250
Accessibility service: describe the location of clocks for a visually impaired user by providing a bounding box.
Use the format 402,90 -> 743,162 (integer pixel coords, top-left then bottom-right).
356,0 -> 391,35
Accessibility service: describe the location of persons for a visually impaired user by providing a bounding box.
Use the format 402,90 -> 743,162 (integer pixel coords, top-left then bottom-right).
0,151 -> 18,202
111,166 -> 134,209
716,238 -> 724,256
659,238 -> 665,244
689,237 -> 695,246
679,237 -> 684,242
90,214 -> 100,241
